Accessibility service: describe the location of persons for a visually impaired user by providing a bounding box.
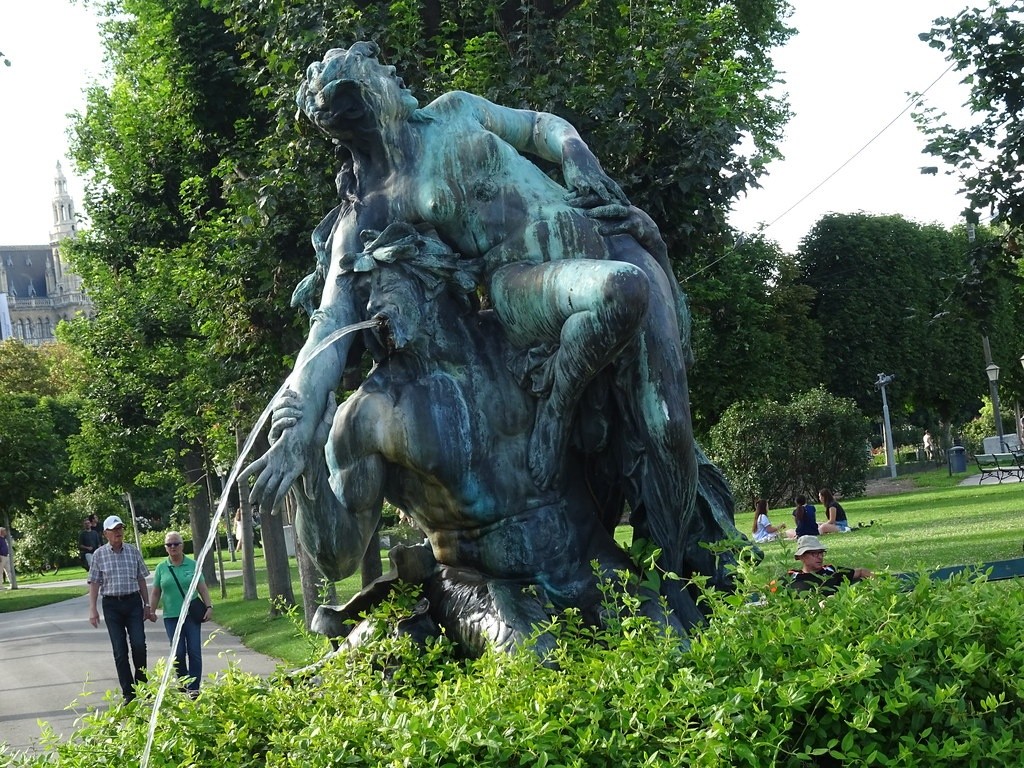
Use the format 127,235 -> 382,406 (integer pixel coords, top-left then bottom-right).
251,507 -> 262,549
778,534 -> 880,607
234,507 -> 245,551
752,500 -> 786,544
817,488 -> 848,534
0,527 -> 16,591
79,514 -> 104,584
87,516 -> 151,707
149,531 -> 213,700
792,495 -> 820,539
235,43 -> 765,670
923,429 -> 934,460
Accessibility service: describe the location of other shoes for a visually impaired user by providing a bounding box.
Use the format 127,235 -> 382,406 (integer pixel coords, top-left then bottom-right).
187,689 -> 200,699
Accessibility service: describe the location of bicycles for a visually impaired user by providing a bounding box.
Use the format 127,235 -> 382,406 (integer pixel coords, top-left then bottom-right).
14,562 -> 58,579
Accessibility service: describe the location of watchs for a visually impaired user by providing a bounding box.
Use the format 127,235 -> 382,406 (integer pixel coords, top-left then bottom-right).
206,605 -> 214,609
144,603 -> 150,607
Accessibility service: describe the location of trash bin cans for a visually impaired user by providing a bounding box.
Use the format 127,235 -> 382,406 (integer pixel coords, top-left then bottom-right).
949,444 -> 968,475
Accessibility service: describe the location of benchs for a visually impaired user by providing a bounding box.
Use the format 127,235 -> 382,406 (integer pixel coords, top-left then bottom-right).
992,453 -> 1024,485
741,557 -> 1024,603
972,454 -> 1001,486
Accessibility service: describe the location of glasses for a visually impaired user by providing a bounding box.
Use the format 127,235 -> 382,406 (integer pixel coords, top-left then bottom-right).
107,526 -> 123,532
164,542 -> 182,547
801,551 -> 827,557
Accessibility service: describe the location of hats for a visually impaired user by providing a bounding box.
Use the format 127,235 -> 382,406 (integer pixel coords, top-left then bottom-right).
103,516 -> 124,531
794,535 -> 826,560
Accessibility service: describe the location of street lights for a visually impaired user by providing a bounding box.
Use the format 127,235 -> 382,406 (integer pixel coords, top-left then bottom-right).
874,372 -> 898,478
979,335 -> 1007,455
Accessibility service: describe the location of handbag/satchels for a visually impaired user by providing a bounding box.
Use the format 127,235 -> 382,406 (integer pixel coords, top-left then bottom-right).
189,597 -> 209,623
